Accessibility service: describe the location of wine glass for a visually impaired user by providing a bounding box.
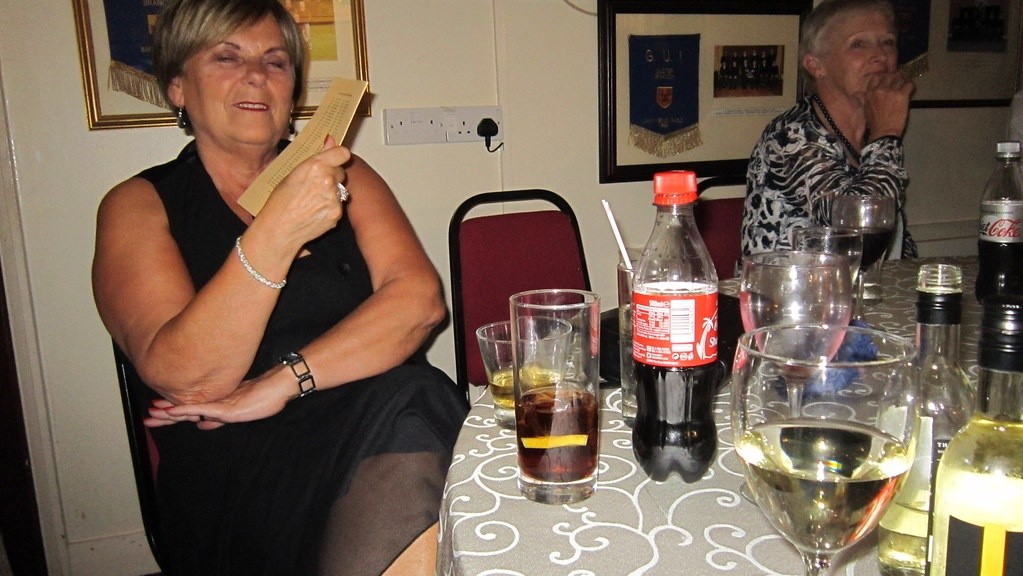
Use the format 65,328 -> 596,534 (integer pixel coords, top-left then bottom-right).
731,324 -> 922,576
742,252 -> 850,505
832,194 -> 893,333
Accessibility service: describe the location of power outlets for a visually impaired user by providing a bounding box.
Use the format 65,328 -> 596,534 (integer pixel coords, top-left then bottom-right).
445,105 -> 503,142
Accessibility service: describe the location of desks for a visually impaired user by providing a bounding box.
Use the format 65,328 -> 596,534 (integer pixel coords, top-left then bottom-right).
442,259 -> 1023,576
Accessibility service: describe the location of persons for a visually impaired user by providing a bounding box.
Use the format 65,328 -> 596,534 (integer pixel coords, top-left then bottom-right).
92,0 -> 470,576
734,0 -> 918,278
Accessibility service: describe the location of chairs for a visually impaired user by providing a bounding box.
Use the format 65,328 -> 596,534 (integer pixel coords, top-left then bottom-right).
448,189 -> 591,400
111,343 -> 165,575
679,176 -> 748,279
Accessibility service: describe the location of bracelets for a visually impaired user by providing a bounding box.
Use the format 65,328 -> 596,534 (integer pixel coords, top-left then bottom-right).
278,352 -> 315,399
236,236 -> 286,288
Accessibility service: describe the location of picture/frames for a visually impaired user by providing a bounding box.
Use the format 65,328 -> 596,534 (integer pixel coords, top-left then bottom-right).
596,0 -> 816,184
70,0 -> 372,131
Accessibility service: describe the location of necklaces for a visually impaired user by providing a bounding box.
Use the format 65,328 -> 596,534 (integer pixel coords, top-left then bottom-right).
812,95 -> 860,163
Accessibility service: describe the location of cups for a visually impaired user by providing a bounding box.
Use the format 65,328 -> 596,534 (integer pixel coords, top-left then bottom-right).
792,227 -> 862,289
510,288 -> 602,504
476,316 -> 574,428
615,263 -> 639,426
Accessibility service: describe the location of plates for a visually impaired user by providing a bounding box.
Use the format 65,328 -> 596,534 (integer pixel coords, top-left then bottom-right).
592,294 -> 787,396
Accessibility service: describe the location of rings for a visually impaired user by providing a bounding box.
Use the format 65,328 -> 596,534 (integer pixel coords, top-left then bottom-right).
337,182 -> 348,202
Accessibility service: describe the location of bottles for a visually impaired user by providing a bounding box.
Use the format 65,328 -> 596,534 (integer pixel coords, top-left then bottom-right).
630,168 -> 720,481
977,142 -> 1023,301
931,294 -> 1023,576
874,264 -> 976,576
